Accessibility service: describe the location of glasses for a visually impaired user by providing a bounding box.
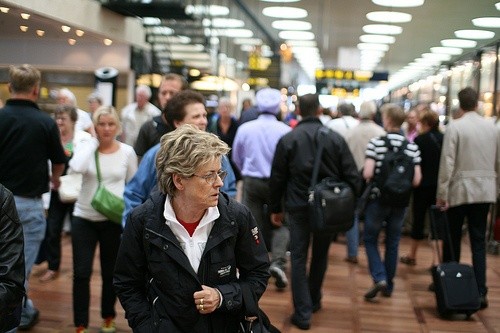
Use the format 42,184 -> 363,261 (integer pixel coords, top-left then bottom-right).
192,171 -> 226,184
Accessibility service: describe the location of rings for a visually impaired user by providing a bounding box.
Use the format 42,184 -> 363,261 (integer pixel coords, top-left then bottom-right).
200,305 -> 203,310
200,298 -> 203,305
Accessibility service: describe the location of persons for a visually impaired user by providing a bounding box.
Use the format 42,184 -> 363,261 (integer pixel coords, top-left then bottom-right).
232,87 -> 293,289
269,94 -> 359,329
0,63 -> 65,283
32,73 -> 445,275
66,104 -> 138,333
39,104 -> 92,281
112,125 -> 272,333
122,90 -> 237,229
0,183 -> 27,332
428,87 -> 500,310
362,103 -> 422,299
400,111 -> 445,271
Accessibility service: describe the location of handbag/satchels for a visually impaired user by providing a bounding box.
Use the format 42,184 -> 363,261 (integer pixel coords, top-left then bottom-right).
58,173 -> 82,202
239,280 -> 282,333
91,185 -> 125,224
308,177 -> 355,232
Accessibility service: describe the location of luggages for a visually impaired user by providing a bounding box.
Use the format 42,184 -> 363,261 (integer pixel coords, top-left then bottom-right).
430,205 -> 480,317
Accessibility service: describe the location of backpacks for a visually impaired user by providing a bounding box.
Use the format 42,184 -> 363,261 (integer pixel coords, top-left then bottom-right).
376,136 -> 414,197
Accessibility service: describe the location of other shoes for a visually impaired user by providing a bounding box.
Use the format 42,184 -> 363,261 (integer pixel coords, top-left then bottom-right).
382,290 -> 390,297
18,312 -> 38,330
345,256 -> 357,264
269,267 -> 288,288
290,314 -> 310,331
39,271 -> 58,283
75,329 -> 90,333
402,257 -> 416,265
365,280 -> 387,299
100,321 -> 116,333
30,261 -> 48,276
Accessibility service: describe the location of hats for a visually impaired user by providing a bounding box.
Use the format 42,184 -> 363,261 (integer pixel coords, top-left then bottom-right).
256,88 -> 282,116
